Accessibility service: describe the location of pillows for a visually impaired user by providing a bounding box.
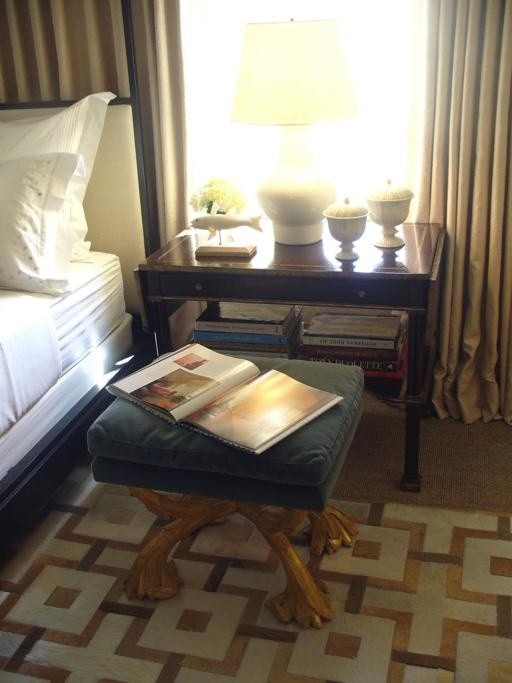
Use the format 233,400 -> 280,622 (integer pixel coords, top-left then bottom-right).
0,92 -> 118,293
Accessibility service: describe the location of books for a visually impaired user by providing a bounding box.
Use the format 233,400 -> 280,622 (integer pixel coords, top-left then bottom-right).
104,343 -> 344,455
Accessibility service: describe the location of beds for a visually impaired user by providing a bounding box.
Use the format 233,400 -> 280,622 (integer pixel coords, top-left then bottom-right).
0,96 -> 157,559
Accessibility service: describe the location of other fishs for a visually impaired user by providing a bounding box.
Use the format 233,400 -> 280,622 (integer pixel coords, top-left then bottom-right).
189,200 -> 263,240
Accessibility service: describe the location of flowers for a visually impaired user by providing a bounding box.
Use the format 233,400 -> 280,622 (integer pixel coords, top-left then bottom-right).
192,177 -> 245,215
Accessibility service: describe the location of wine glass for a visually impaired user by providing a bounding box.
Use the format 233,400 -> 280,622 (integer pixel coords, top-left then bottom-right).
322,195 -> 371,262
367,180 -> 414,249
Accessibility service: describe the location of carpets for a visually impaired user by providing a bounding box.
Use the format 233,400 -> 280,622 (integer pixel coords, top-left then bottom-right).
0,481 -> 510,683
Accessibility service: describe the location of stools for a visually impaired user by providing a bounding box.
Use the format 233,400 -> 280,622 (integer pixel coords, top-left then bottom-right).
86,354 -> 364,629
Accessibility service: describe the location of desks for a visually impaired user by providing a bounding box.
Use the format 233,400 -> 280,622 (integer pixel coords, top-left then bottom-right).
139,221 -> 447,492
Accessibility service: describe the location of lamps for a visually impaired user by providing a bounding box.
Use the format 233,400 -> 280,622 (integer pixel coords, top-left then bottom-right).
229,22 -> 356,246
